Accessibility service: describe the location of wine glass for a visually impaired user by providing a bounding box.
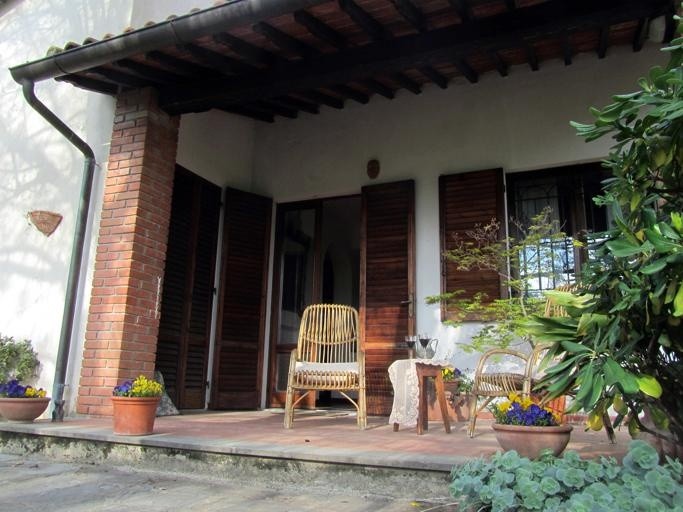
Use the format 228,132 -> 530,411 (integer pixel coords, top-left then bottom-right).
405,336 -> 417,359
419,333 -> 430,359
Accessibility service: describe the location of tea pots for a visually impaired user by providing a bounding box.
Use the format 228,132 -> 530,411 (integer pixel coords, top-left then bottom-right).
419,338 -> 438,359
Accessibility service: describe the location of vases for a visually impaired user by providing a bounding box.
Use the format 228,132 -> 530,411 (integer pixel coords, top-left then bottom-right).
444,381 -> 460,391
493,423 -> 573,462
111,395 -> 161,436
0,394 -> 51,424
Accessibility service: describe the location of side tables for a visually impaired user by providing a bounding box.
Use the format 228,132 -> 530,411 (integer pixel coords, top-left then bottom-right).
388,358 -> 452,436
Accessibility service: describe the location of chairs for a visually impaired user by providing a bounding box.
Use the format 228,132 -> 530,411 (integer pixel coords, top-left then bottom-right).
467,283 -> 617,444
284,303 -> 367,431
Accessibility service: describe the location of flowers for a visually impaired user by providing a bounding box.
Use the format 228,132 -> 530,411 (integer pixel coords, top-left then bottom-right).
485,392 -> 562,426
1,378 -> 48,397
111,374 -> 164,398
440,366 -> 462,382
